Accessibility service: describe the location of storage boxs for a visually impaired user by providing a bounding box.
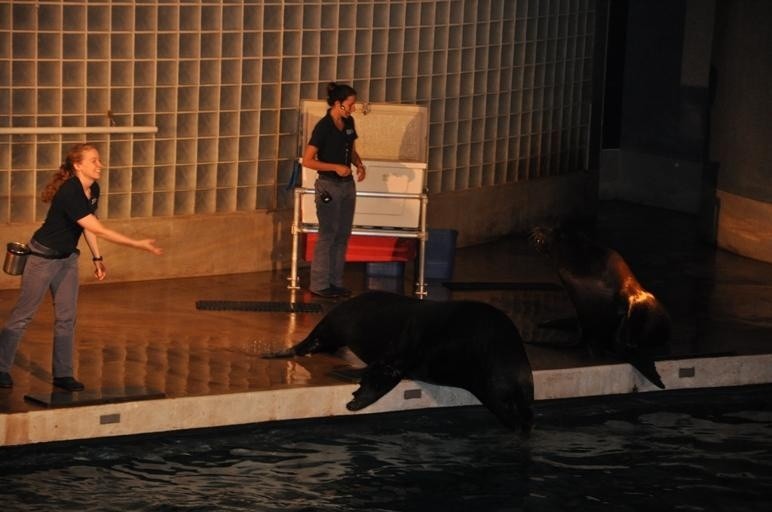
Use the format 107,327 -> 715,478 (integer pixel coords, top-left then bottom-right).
365,221 -> 458,280
300,97 -> 431,227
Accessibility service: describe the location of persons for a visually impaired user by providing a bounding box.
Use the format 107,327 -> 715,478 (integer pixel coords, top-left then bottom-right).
0,145 -> 165,392
303,82 -> 366,298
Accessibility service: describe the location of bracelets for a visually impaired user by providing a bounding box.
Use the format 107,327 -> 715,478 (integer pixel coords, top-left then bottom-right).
93,256 -> 102,261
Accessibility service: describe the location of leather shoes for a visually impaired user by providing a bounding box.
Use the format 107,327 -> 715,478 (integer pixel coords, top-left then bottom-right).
52,375 -> 85,392
0,372 -> 14,388
312,283 -> 352,298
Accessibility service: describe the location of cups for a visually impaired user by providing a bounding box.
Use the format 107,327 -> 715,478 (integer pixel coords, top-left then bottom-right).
2,242 -> 29,276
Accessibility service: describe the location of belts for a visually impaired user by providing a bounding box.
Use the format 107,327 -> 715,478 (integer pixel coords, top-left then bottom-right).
30,236 -> 79,256
317,172 -> 353,182
